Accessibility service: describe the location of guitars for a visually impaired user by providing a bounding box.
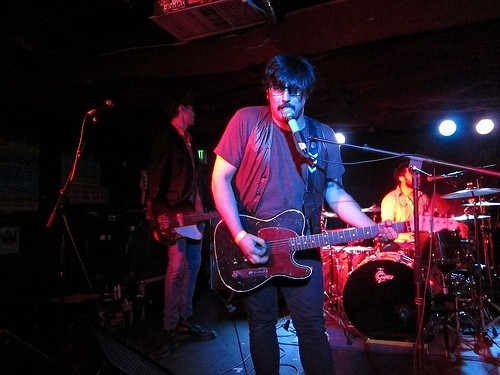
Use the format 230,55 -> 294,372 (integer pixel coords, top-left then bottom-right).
151,205 -> 239,246
212,209 -> 469,293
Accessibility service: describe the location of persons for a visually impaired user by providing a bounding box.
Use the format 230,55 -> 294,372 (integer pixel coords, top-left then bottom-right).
382,165 -> 432,261
211,54 -> 399,375
142,95 -> 216,361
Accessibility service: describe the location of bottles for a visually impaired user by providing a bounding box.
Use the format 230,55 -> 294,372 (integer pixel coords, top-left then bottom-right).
113,283 -> 121,300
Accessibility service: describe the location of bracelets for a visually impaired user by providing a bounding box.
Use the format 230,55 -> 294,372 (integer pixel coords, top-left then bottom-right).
234,230 -> 247,245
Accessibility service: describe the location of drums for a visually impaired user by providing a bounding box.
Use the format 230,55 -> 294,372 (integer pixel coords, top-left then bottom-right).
333,246 -> 376,302
430,228 -> 462,262
421,264 -> 456,295
343,252 -> 431,341
439,240 -> 473,271
320,245 -> 344,299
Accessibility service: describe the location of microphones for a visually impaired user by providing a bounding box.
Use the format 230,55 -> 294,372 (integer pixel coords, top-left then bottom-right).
282,106 -> 307,152
85,99 -> 114,115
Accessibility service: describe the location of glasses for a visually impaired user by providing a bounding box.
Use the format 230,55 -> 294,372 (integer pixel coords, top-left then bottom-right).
268,84 -> 308,96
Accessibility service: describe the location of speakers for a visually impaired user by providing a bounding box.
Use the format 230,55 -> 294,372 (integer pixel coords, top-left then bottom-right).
53,314 -> 178,375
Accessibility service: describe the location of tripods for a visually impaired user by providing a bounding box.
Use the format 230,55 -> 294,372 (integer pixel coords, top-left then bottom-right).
439,172 -> 500,346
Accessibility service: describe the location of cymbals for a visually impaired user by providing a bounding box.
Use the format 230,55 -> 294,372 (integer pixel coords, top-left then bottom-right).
447,214 -> 492,220
439,187 -> 500,199
463,201 -> 500,206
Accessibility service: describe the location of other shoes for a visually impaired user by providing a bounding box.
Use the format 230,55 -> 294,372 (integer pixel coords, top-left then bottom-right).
151,333 -> 180,359
178,323 -> 217,338
